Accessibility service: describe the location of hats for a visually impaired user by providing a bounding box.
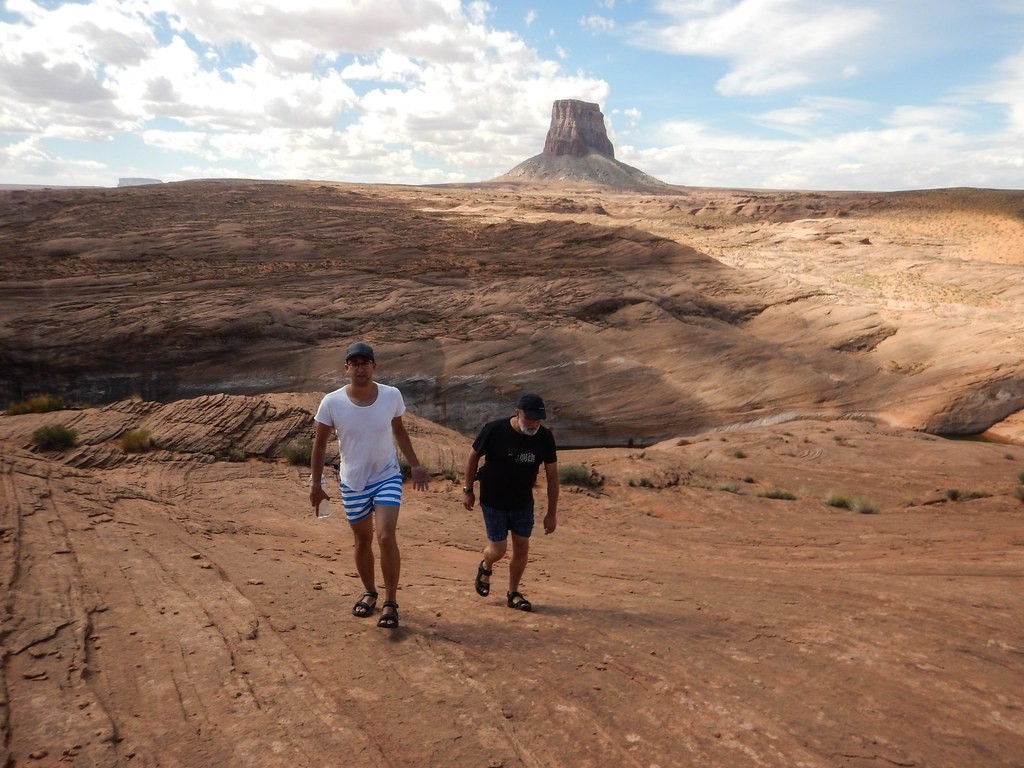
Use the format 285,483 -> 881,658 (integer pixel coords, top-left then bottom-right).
517,393 -> 546,421
346,342 -> 374,364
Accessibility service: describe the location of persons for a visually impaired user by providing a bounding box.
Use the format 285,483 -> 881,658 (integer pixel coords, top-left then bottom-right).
309,342 -> 429,629
463,393 -> 559,610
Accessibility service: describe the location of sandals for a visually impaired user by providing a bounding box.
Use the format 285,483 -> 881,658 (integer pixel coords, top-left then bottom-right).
352,592 -> 378,617
377,601 -> 399,628
475,560 -> 492,597
507,591 -> 531,610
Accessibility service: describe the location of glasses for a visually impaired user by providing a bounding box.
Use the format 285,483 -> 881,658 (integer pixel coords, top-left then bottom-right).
348,360 -> 373,370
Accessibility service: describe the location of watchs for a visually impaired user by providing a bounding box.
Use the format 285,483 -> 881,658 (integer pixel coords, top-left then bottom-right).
463,487 -> 473,493
